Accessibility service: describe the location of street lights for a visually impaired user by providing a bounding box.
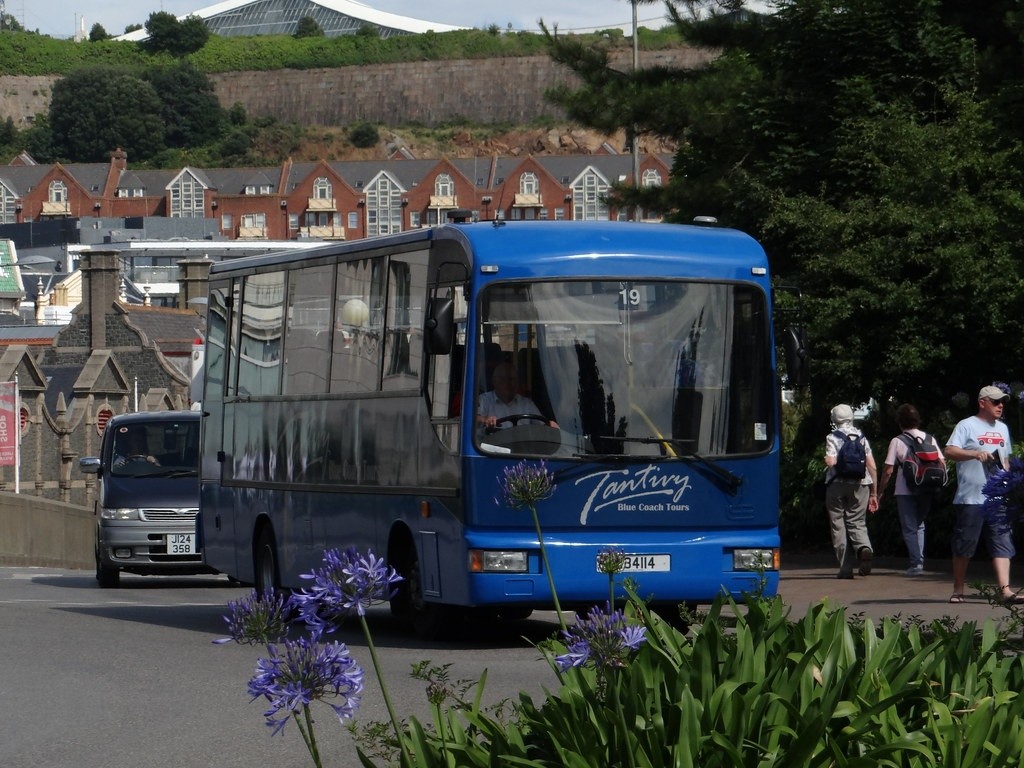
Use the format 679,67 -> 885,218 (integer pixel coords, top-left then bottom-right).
340,299 -> 368,480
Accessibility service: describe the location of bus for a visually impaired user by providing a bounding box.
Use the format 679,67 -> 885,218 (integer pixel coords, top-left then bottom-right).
196,213 -> 806,631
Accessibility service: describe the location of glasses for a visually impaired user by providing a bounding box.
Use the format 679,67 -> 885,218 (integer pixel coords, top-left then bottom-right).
991,400 -> 1005,405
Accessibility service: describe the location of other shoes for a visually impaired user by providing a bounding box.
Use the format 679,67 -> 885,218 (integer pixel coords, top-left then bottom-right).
1004,597 -> 1024,604
837,572 -> 854,578
950,595 -> 964,603
859,550 -> 873,576
907,565 -> 923,575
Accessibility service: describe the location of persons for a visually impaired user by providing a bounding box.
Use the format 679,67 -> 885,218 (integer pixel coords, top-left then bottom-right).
473,362 -> 561,450
823,403 -> 879,580
944,385 -> 1024,605
872,402 -> 949,577
113,431 -> 163,474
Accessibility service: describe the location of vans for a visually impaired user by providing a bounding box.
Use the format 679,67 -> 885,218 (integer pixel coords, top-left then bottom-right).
271,409 -> 334,492
78,412 -> 247,587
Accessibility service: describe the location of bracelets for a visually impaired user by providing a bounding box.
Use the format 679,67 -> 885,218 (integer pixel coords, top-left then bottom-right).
870,493 -> 878,497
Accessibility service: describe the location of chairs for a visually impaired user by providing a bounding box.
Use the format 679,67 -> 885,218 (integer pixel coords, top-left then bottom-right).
290,329 -> 543,400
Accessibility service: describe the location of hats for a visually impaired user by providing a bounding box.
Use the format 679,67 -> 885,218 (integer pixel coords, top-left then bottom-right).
979,386 -> 1010,401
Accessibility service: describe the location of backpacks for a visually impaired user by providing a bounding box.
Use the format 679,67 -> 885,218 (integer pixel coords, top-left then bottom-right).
896,431 -> 948,493
831,430 -> 866,479
